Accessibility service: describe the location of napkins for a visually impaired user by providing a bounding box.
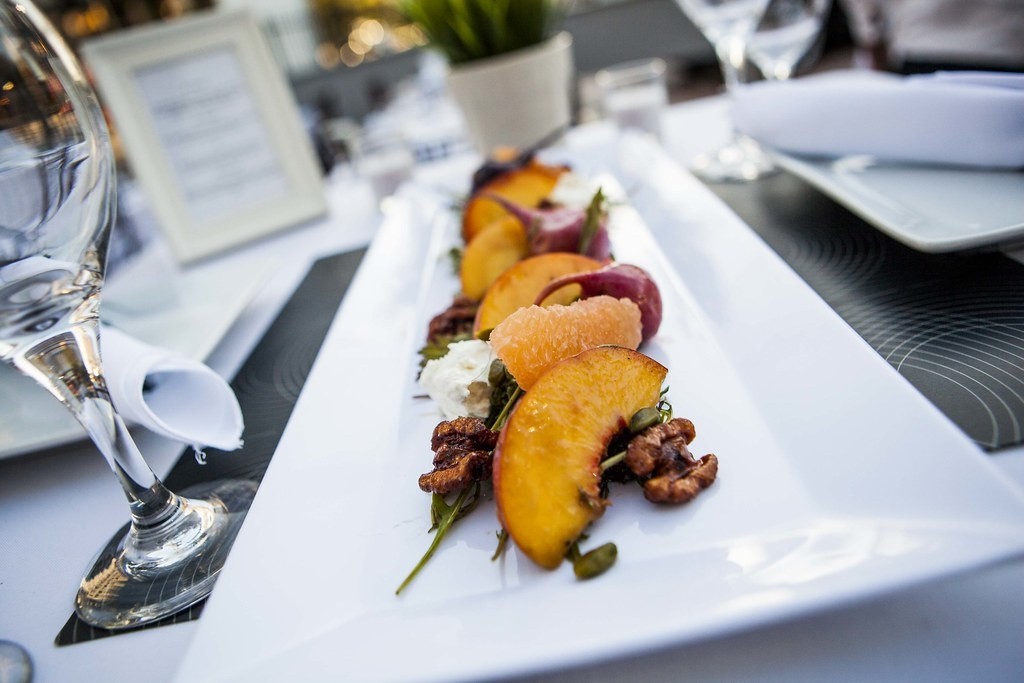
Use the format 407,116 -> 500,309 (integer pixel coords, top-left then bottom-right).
729,66 -> 1024,169
97,315 -> 248,463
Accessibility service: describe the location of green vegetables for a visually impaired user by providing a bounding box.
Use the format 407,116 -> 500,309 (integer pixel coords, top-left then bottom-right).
394,186 -> 672,594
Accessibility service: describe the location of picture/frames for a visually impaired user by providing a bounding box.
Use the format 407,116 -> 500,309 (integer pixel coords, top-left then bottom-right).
79,3 -> 334,265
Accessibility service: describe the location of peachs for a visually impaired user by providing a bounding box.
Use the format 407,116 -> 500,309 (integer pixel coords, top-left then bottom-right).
457,159 -> 670,569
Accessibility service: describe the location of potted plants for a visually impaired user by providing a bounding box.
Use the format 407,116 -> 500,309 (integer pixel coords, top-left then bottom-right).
396,0 -> 575,163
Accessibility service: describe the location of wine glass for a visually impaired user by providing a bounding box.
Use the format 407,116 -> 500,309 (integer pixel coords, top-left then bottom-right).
675,0 -> 778,179
747,0 -> 821,80
0,0 -> 258,631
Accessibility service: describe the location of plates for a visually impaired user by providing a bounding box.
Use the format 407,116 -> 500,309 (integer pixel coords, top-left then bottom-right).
0,282 -> 241,458
758,141 -> 1024,252
177,123 -> 1024,683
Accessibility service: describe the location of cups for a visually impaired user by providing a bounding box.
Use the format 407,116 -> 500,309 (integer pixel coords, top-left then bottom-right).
596,59 -> 665,145
